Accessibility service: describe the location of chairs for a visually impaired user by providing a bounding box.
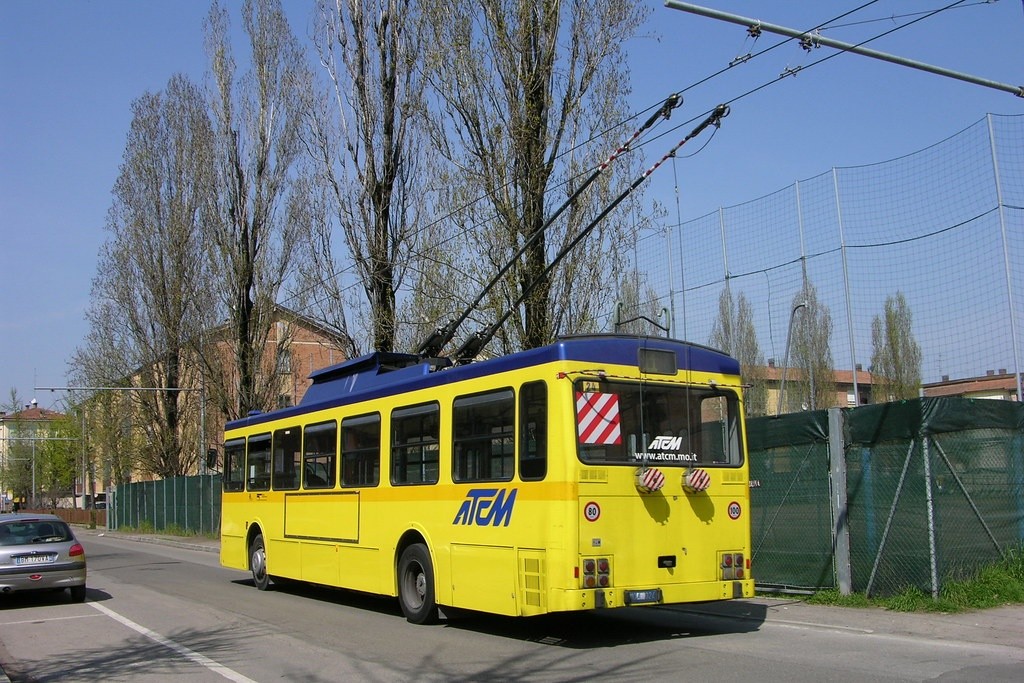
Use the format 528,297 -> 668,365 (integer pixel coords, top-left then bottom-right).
0,526 -> 10,537
39,524 -> 54,536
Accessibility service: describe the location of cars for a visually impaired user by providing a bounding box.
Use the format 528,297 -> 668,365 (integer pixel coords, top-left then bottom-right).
0,513 -> 87,605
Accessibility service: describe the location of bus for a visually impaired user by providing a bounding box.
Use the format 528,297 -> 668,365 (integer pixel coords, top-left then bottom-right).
206,330 -> 755,624
